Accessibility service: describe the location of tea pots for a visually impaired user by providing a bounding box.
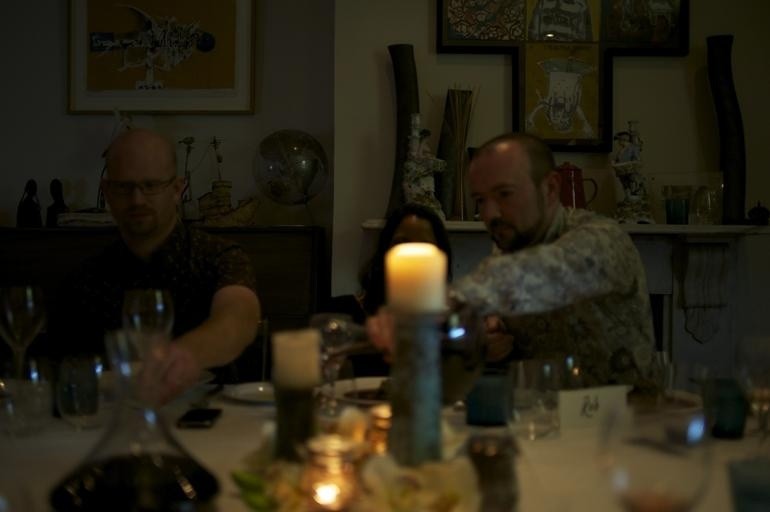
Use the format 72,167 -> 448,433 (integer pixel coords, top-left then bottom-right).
554,161 -> 597,211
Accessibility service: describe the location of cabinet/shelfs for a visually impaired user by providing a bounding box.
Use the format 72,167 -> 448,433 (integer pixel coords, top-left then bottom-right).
0,225 -> 329,380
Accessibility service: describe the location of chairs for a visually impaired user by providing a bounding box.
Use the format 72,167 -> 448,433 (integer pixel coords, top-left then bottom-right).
650,291 -> 665,353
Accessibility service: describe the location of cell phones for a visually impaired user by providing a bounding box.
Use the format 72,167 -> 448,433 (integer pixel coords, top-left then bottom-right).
176,408 -> 222,429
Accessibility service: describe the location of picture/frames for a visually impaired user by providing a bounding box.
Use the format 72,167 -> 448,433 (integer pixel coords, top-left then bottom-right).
435,0 -> 691,153
66,0 -> 254,115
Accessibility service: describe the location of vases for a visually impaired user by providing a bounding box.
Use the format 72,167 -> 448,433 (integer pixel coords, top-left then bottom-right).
704,33 -> 747,225
384,43 -> 420,219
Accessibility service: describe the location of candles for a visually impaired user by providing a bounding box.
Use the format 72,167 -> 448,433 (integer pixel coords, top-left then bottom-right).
272,327 -> 319,392
385,241 -> 447,314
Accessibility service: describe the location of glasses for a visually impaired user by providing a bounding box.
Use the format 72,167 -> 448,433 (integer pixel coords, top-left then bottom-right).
104,176 -> 177,197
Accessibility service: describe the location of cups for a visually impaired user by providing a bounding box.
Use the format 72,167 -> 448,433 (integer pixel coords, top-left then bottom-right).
696,184 -> 724,224
663,185 -> 689,225
505,361 -> 560,438
692,361 -> 752,440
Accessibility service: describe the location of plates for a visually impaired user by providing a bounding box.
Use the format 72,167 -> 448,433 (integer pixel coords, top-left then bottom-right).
319,375 -> 395,406
222,380 -> 281,404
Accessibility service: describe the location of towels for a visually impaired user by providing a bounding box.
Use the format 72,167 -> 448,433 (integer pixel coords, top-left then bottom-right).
683,246 -> 729,344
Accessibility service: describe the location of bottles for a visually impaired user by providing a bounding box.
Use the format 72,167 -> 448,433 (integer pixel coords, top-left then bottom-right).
48,338 -> 221,511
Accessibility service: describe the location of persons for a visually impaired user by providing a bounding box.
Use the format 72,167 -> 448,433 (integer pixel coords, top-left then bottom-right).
362,132 -> 658,396
0,125 -> 263,413
312,199 -> 456,387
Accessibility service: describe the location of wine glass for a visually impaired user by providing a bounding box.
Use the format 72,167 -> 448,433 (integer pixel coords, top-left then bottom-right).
1,283 -> 47,400
310,313 -> 357,419
121,287 -> 176,415
595,389 -> 711,512
